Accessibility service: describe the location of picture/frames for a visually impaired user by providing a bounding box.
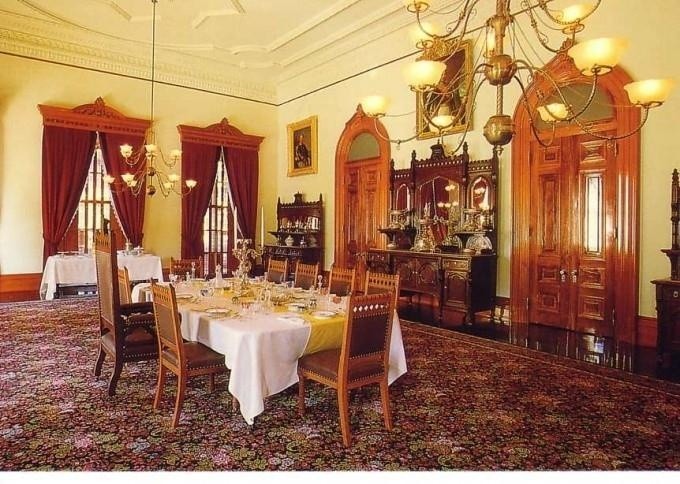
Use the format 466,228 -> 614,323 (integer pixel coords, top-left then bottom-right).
286,115 -> 318,178
414,36 -> 476,141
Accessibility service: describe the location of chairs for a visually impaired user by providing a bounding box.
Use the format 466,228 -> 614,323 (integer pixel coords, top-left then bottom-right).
327,264 -> 358,298
149,277 -> 240,428
295,260 -> 320,289
295,285 -> 397,446
266,255 -> 290,284
169,255 -> 206,281
89,227 -> 185,398
364,267 -> 401,309
115,264 -> 134,305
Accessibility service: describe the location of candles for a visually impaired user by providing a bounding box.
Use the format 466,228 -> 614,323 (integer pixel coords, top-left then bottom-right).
233,204 -> 237,250
260,203 -> 264,250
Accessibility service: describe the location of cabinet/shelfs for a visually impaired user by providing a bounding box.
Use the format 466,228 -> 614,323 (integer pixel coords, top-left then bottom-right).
650,165 -> 679,368
366,240 -> 498,330
257,191 -> 324,281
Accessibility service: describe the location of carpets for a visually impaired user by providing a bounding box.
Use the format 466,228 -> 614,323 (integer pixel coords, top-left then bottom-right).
0,292 -> 679,470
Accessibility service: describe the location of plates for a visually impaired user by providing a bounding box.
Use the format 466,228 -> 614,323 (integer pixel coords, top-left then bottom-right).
309,309 -> 337,318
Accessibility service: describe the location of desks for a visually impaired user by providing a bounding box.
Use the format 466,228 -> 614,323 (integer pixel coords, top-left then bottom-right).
48,252 -> 162,301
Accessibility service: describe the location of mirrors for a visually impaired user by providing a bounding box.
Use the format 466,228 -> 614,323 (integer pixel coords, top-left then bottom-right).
387,154 -> 413,227
413,137 -> 466,252
466,141 -> 496,229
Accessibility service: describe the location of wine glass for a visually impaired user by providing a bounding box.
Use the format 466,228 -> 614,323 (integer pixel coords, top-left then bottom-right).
169,266 -> 347,315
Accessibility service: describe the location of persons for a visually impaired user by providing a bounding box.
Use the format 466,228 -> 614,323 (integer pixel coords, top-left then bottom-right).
295,134 -> 311,168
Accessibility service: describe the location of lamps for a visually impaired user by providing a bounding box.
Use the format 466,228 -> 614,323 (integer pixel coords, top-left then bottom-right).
98,0 -> 204,201
357,0 -> 676,163
